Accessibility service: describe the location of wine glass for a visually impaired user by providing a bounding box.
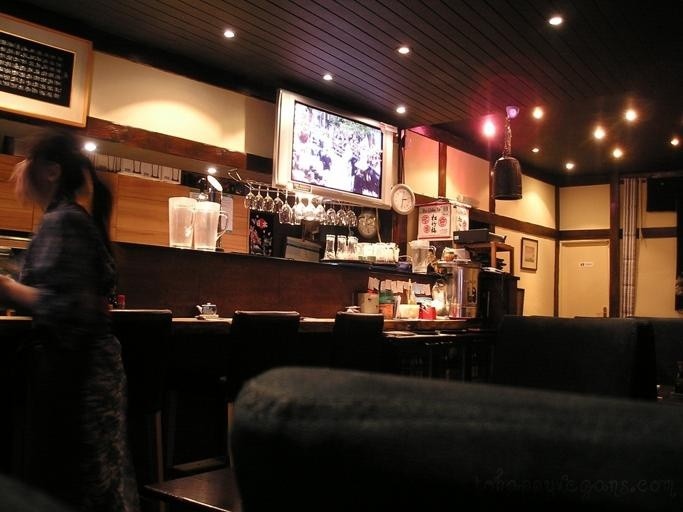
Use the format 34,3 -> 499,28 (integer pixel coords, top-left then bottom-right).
244,180 -> 358,227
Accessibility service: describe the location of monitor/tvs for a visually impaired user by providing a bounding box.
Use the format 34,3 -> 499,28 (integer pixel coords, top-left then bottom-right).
272,88 -> 394,211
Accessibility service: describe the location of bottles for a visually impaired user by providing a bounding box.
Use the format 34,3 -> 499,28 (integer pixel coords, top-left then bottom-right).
444,252 -> 456,262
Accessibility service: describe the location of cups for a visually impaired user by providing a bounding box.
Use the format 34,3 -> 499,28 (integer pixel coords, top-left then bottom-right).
409,240 -> 436,274
168,196 -> 230,251
324,234 -> 400,264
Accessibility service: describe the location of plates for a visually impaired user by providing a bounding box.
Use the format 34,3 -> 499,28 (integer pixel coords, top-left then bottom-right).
357,211 -> 381,240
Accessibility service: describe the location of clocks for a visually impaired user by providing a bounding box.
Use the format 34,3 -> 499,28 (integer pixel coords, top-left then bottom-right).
390,184 -> 417,217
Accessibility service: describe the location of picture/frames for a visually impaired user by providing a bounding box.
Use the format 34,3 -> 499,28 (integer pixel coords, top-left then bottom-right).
519,236 -> 539,272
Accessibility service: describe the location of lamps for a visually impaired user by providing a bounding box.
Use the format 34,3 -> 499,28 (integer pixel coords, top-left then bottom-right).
488,105 -> 523,202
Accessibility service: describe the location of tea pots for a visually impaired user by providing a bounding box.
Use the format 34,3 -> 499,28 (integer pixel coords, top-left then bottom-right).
196,302 -> 217,316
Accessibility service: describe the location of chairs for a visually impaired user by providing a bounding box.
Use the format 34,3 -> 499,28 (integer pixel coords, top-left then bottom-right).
0,303 -> 683,512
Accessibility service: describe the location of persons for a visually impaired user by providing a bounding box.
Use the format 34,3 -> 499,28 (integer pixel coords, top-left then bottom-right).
0,134 -> 141,511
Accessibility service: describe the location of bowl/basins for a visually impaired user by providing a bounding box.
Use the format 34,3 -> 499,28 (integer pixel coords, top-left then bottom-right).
399,304 -> 420,319
